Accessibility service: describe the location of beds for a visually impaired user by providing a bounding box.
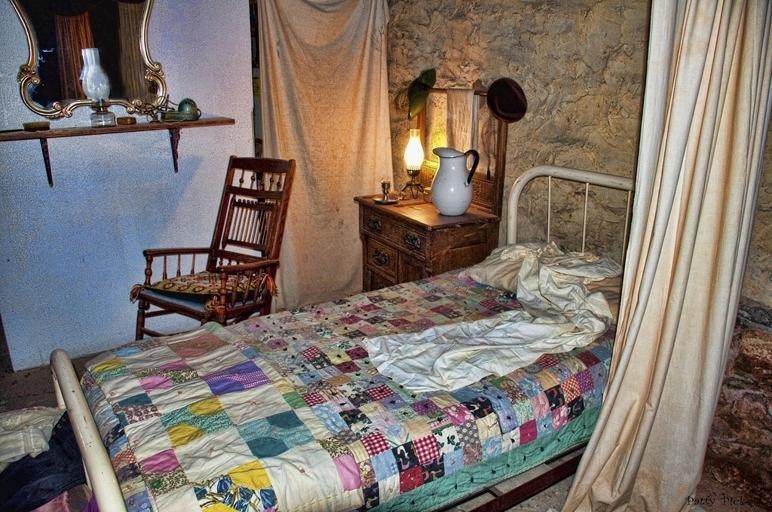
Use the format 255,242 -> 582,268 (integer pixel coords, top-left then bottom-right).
48,166 -> 636,511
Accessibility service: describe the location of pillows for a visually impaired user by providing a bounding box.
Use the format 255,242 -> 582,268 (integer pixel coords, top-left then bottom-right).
458,245 -> 622,326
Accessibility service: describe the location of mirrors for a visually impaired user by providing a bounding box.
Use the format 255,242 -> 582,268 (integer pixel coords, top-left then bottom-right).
9,0 -> 167,120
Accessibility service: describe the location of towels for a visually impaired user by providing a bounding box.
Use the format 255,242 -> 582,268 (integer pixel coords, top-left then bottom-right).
444,87 -> 479,171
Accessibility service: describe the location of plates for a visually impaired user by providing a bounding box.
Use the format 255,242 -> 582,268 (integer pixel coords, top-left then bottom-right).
370,196 -> 399,205
21,121 -> 51,132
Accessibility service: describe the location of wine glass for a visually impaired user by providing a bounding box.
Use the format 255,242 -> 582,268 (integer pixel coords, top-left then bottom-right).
377,176 -> 390,202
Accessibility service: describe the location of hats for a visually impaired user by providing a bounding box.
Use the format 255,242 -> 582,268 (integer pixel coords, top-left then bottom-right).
487,77 -> 527,123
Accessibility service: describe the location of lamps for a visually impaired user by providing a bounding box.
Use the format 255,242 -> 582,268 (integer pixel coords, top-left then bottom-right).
399,128 -> 425,200
78,47 -> 117,128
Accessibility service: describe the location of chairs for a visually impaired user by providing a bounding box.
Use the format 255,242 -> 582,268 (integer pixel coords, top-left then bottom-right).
135,156 -> 296,342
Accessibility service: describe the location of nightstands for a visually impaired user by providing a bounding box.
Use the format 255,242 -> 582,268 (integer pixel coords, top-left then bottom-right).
353,87 -> 507,292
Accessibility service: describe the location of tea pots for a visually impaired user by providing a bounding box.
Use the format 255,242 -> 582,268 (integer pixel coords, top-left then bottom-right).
427,147 -> 479,219
